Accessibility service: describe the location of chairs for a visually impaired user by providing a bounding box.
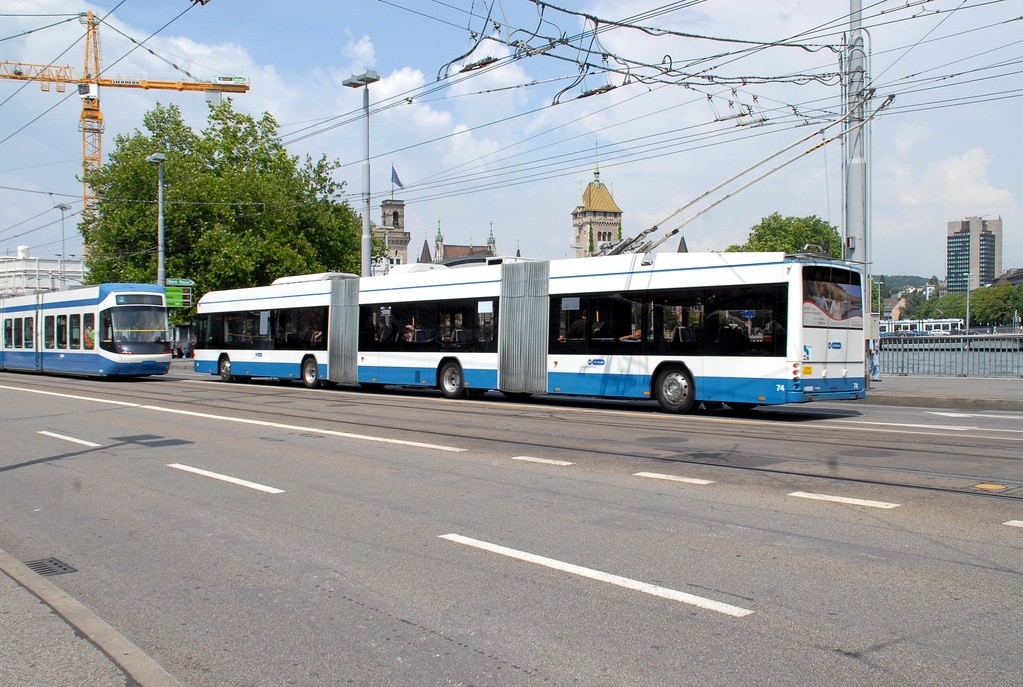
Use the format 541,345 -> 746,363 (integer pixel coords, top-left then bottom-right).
669,323 -> 751,343
285,333 -> 298,342
378,327 -> 480,342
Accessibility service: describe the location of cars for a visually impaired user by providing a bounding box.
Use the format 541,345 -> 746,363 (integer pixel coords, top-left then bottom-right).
879,327 -> 979,337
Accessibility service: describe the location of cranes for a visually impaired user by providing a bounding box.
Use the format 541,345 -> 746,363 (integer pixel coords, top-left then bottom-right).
1,9 -> 250,287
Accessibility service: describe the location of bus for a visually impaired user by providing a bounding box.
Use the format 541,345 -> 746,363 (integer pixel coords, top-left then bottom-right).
191,226 -> 874,417
0,281 -> 174,382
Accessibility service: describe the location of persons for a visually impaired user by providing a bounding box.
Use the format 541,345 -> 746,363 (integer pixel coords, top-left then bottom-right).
619,329 -> 641,342
990,325 -> 999,335
171,332 -> 197,359
374,320 -> 413,343
869,337 -> 884,382
816,287 -> 843,321
557,310 -> 587,341
84,325 -> 129,350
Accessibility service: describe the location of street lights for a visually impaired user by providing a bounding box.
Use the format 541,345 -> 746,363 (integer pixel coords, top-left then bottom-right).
874,281 -> 884,314
145,152 -> 169,286
963,272 -> 974,349
341,70 -> 383,277
